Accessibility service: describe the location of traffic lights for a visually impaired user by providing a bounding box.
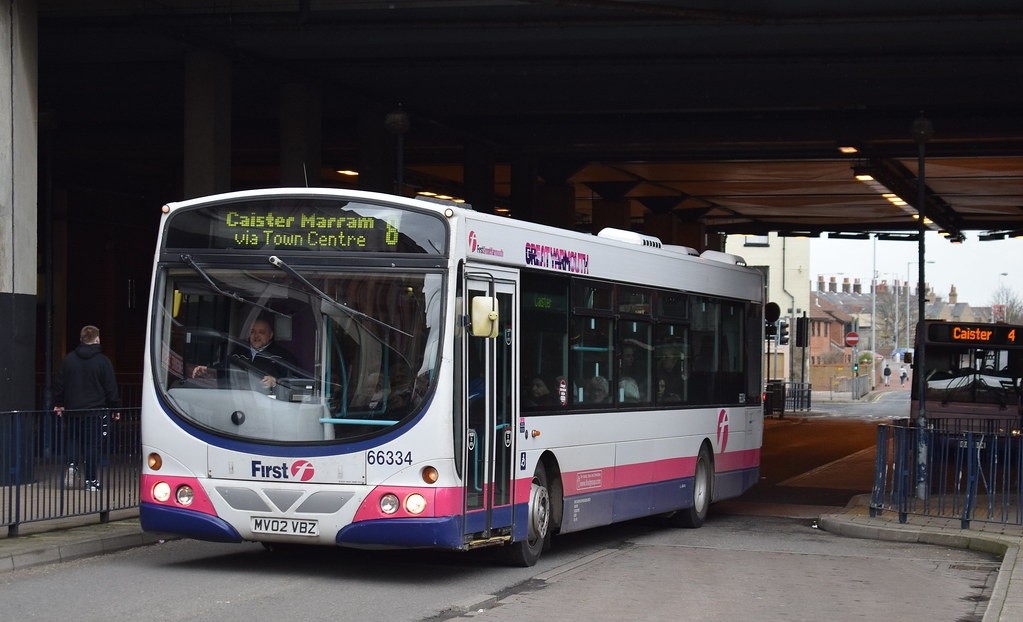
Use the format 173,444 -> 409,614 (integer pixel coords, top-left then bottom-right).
780,322 -> 789,345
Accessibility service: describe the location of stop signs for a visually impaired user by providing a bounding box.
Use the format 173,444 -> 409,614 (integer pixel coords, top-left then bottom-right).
846,332 -> 859,346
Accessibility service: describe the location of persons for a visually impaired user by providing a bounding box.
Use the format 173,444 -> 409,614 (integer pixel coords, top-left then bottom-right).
521,374 -> 561,408
884,364 -> 891,387
899,365 -> 908,388
654,375 -> 682,402
584,376 -> 613,404
191,317 -> 300,396
618,344 -> 645,386
53,325 -> 121,491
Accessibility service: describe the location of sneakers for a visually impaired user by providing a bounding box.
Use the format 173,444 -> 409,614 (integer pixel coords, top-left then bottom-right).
64,463 -> 77,488
85,480 -> 102,491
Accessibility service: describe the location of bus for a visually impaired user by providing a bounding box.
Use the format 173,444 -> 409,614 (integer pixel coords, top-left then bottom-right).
139,187 -> 765,569
909,318 -> 1022,448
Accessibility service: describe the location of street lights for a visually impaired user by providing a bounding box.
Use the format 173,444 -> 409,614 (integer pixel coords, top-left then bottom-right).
998,272 -> 1008,321
907,261 -> 936,381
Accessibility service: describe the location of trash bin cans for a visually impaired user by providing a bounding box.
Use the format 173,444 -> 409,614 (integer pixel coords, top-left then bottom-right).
768,379 -> 787,412
